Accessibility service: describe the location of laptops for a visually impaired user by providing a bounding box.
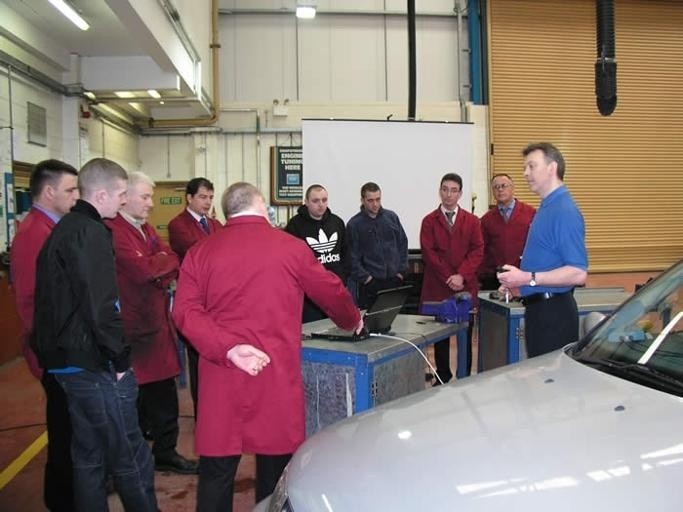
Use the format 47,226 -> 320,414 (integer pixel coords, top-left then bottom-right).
311,285 -> 413,342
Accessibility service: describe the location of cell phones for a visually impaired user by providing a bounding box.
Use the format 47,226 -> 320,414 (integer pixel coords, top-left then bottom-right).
496,265 -> 510,274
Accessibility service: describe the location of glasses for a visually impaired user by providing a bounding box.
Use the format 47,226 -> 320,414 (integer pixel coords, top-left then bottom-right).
494,184 -> 507,189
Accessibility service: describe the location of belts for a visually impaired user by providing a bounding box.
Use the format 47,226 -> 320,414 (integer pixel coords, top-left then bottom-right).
522,292 -> 558,305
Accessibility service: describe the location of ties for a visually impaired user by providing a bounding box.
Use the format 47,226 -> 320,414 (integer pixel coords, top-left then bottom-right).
501,209 -> 508,223
199,219 -> 208,233
445,212 -> 454,226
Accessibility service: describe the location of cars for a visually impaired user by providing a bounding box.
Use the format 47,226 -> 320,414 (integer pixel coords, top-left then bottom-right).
250,251 -> 682,511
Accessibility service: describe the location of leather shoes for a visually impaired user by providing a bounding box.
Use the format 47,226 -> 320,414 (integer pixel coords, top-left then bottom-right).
154,454 -> 198,474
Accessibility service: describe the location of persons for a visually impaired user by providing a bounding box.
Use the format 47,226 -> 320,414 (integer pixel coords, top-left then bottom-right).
27,159 -> 163,511
496,140 -> 592,364
418,172 -> 486,387
103,169 -> 200,477
479,174 -> 538,290
7,159 -> 79,509
346,182 -> 409,313
282,174 -> 347,324
170,181 -> 364,510
168,177 -> 224,419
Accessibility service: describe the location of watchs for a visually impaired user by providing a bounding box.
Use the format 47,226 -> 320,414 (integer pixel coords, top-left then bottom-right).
528,270 -> 539,288
